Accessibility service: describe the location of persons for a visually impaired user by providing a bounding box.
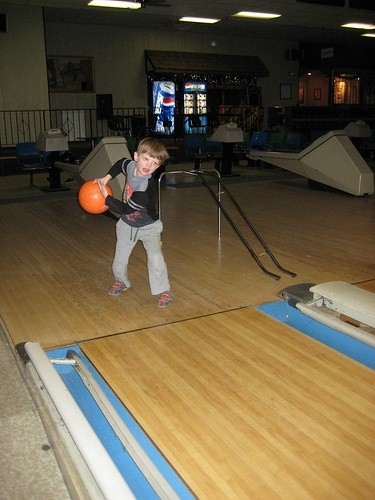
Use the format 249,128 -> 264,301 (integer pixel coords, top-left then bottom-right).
93,137 -> 173,307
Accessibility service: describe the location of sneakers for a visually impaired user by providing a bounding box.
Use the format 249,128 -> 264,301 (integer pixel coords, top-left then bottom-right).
108,279 -> 128,296
158,289 -> 174,307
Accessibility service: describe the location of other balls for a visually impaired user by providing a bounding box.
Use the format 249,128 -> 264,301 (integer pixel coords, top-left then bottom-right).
78,179 -> 113,215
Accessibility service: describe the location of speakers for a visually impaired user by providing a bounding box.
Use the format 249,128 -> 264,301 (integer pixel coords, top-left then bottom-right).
96,94 -> 113,116
285,49 -> 300,60
